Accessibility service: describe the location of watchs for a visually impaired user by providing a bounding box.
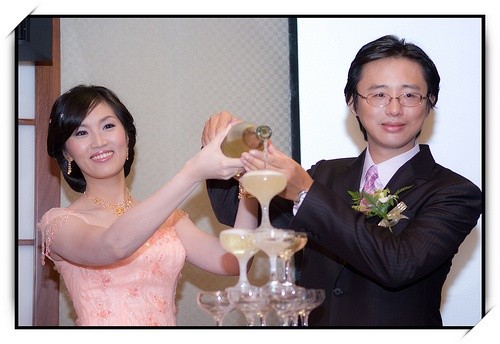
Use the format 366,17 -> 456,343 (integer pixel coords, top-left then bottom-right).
294,189 -> 310,206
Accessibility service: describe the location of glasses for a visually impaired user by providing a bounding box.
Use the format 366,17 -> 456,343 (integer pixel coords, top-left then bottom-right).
356,89 -> 428,108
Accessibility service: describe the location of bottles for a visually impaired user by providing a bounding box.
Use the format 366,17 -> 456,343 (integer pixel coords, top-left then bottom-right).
200,122 -> 272,158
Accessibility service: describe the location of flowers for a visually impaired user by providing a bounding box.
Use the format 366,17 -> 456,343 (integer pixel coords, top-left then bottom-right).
346,186 -> 416,233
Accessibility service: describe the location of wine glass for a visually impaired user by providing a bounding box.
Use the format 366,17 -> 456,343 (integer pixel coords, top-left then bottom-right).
219,231 -> 308,289
239,171 -> 288,232
199,283 -> 325,326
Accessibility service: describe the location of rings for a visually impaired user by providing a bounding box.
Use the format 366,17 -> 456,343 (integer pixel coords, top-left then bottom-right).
235,171 -> 241,177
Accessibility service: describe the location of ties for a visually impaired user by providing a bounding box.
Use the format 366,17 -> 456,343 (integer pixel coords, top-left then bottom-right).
357,164 -> 378,215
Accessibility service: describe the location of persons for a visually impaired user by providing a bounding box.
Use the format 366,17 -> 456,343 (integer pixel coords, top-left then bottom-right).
37,84 -> 259,326
202,35 -> 482,327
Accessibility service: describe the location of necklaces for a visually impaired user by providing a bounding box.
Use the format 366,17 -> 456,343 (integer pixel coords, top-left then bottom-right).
83,191 -> 133,215
